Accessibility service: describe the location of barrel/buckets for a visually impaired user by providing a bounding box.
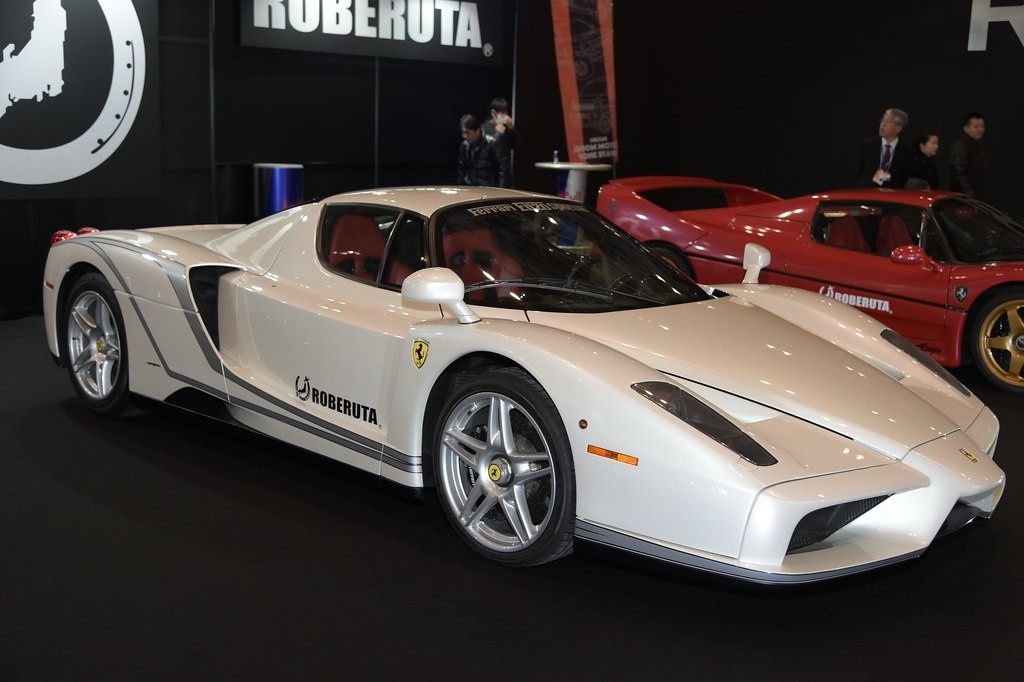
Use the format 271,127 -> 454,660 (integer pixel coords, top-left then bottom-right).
253,162 -> 303,220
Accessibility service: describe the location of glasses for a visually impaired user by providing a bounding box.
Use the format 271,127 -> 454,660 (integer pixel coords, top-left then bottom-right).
969,124 -> 985,130
877,118 -> 896,125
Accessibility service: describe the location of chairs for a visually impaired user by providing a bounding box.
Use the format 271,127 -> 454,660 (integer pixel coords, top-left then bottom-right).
827,217 -> 871,254
441,221 -> 524,302
328,214 -> 413,286
877,215 -> 916,258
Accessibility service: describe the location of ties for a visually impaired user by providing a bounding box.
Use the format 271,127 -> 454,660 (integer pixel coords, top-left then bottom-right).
881,144 -> 891,172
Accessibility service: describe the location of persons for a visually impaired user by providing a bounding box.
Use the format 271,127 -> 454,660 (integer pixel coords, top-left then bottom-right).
852,109 -> 940,191
458,97 -> 525,188
947,113 -> 989,199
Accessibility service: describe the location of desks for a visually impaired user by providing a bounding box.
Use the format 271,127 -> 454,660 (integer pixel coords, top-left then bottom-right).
535,162 -> 611,248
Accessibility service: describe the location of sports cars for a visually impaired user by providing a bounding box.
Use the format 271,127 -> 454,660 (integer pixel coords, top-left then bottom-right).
41,184 -> 1008,587
594,174 -> 1024,399
